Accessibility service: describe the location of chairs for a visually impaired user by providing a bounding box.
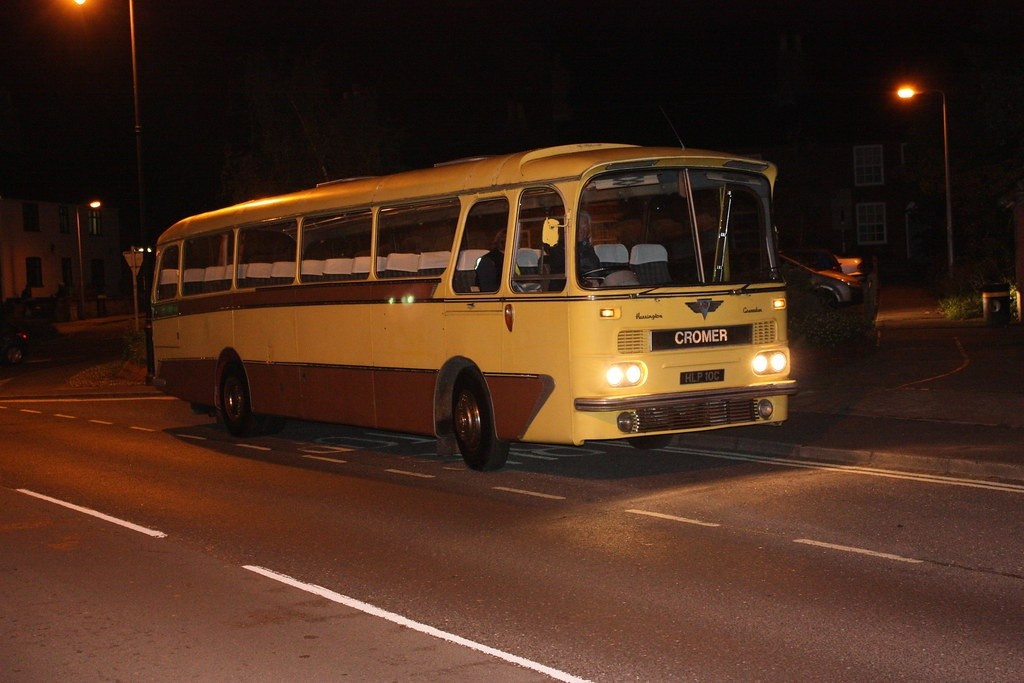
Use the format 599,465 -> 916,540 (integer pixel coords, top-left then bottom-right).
630,244 -> 672,283
245,263 -> 271,287
183,268 -> 204,295
300,260 -> 322,282
457,250 -> 490,292
272,262 -> 295,285
419,250 -> 451,276
351,256 -> 382,280
204,266 -> 226,293
384,252 -> 418,279
322,258 -> 353,281
594,244 -> 629,275
159,268 -> 178,299
224,263 -> 247,291
515,248 -> 539,282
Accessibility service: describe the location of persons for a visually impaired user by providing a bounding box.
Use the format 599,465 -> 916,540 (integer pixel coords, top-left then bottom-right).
21,284 -> 32,297
538,209 -> 601,291
474,229 -> 506,293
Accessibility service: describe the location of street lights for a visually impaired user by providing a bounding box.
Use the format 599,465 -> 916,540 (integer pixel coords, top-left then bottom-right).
897,86 -> 955,282
75,198 -> 102,322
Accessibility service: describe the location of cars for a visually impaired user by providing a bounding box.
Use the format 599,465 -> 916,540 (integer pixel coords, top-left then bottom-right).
777,247 -> 867,313
0,317 -> 34,366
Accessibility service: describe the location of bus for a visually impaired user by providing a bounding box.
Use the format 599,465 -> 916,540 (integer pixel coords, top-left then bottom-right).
146,140 -> 801,473
